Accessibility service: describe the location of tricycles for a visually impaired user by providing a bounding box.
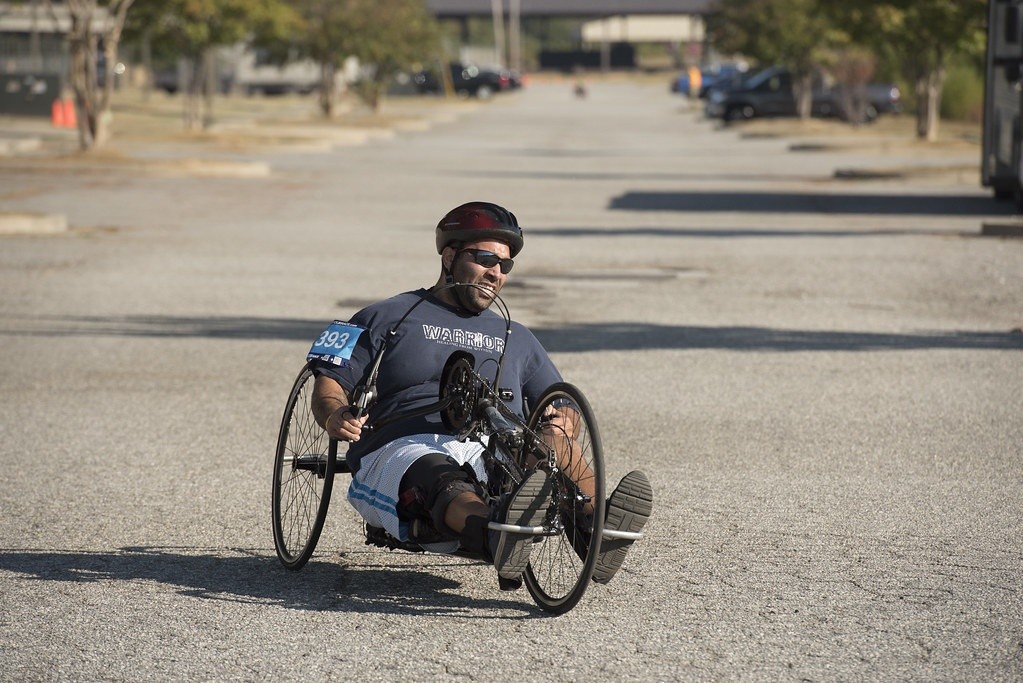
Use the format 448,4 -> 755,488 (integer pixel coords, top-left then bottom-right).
269,283 -> 645,617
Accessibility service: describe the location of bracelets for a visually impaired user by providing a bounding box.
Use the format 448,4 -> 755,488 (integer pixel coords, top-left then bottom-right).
325,415 -> 331,431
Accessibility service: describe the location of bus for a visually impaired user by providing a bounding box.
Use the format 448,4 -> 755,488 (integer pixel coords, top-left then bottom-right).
980,1 -> 1023,204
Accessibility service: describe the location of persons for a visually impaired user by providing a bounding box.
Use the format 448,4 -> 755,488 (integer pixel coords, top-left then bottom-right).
311,201 -> 653,584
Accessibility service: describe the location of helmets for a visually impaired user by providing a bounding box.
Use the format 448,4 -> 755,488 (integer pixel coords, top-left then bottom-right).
436,202 -> 523,258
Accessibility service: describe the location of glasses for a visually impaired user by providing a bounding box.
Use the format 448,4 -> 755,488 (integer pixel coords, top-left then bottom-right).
458,248 -> 514,275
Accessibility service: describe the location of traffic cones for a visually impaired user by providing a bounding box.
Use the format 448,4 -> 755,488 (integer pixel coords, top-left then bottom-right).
49,96 -> 78,129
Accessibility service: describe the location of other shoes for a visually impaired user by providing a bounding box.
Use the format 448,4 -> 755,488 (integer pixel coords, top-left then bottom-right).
574,470 -> 653,584
488,470 -> 552,580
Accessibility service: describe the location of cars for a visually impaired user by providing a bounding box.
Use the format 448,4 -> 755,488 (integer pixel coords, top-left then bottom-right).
380,63 -> 523,99
672,56 -> 763,98
86,47 -> 180,91
705,63 -> 901,123
0,72 -> 73,115
